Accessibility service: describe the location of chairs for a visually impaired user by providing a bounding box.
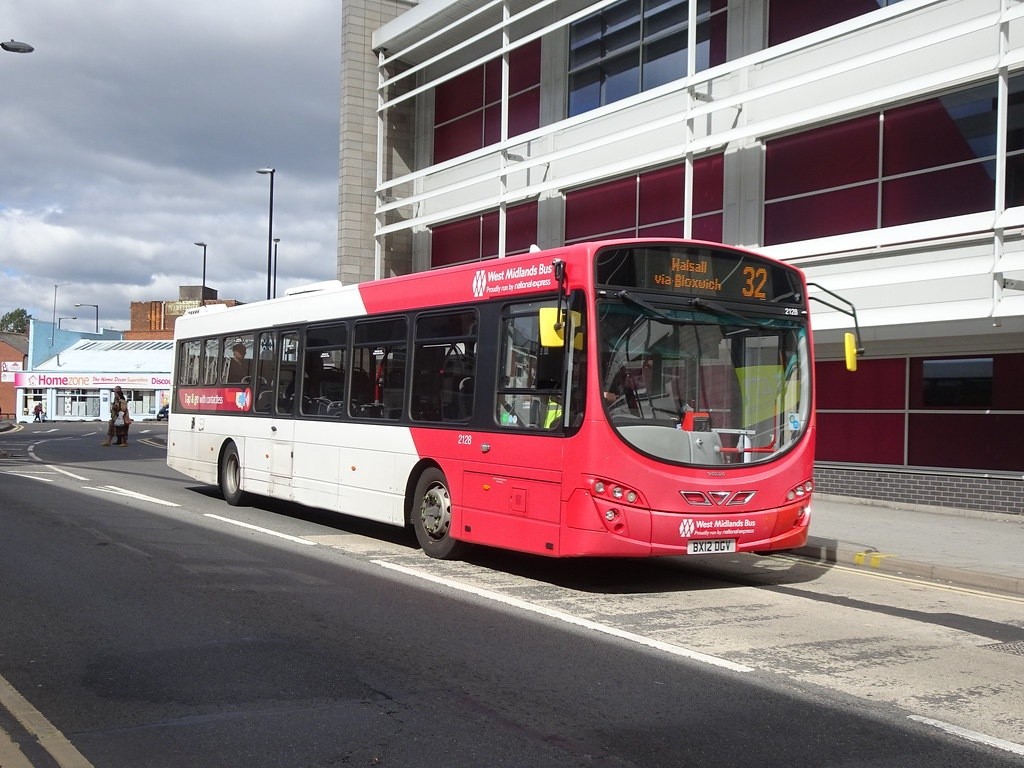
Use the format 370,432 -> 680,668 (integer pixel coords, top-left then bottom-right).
241,374 -> 400,417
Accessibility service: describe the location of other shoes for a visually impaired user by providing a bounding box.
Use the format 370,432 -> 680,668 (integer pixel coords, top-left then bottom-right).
113,442 -> 121,445
101,442 -> 111,446
119,444 -> 126,447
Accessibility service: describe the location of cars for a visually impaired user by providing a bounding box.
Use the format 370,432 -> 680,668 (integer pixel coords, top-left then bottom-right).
157,404 -> 169,421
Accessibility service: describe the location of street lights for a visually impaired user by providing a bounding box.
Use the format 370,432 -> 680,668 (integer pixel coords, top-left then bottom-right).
74,302 -> 99,334
193,241 -> 209,308
255,165 -> 276,299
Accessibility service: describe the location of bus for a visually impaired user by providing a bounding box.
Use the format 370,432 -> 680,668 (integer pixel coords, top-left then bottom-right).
166,237 -> 866,565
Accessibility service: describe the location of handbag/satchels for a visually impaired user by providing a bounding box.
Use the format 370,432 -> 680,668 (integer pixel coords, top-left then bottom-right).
114,411 -> 125,427
33,411 -> 36,414
123,415 -> 131,425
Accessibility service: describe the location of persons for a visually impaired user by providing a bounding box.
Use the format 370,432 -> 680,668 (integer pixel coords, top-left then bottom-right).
34,403 -> 42,421
101,386 -> 129,447
225,344 -> 252,383
